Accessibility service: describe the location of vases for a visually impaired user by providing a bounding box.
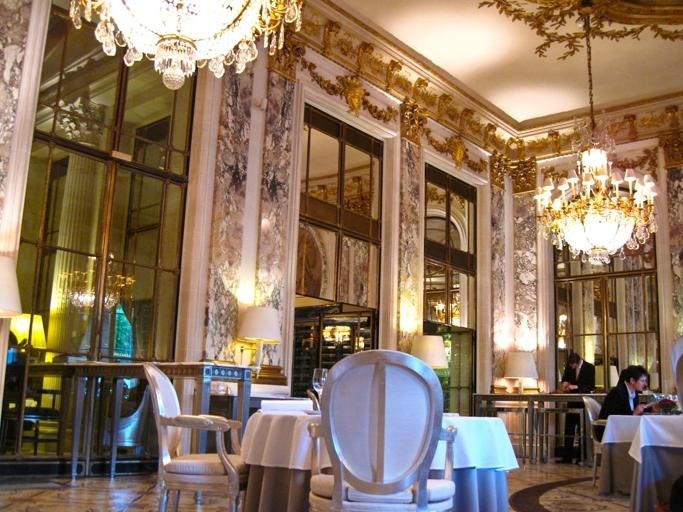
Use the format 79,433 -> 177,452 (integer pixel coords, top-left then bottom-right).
662,410 -> 671,415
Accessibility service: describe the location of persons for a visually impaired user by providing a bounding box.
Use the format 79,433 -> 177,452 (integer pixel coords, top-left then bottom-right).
592,365 -> 649,442
554,353 -> 596,465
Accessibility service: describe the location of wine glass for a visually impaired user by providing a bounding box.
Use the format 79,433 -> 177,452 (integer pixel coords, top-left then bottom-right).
313,366 -> 331,414
654,393 -> 680,413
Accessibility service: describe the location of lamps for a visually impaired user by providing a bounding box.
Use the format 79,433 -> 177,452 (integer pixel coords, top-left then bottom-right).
237,308 -> 282,379
543,0 -> 658,267
503,352 -> 538,395
70,0 -> 302,91
63,254 -> 134,319
595,366 -> 619,388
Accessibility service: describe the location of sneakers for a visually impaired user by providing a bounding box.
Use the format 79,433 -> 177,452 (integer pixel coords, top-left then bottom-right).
555,457 -> 573,464
573,459 -> 585,465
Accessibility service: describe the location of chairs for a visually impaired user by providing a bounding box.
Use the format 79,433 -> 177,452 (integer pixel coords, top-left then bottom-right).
143,362 -> 250,512
308,350 -> 457,512
583,396 -> 608,486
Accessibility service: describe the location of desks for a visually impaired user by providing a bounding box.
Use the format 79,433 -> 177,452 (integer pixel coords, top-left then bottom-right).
473,393 -> 608,464
0,363 -> 112,457
62,361 -> 252,480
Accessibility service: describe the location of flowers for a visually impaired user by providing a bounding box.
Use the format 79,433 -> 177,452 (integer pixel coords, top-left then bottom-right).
659,399 -> 677,410
